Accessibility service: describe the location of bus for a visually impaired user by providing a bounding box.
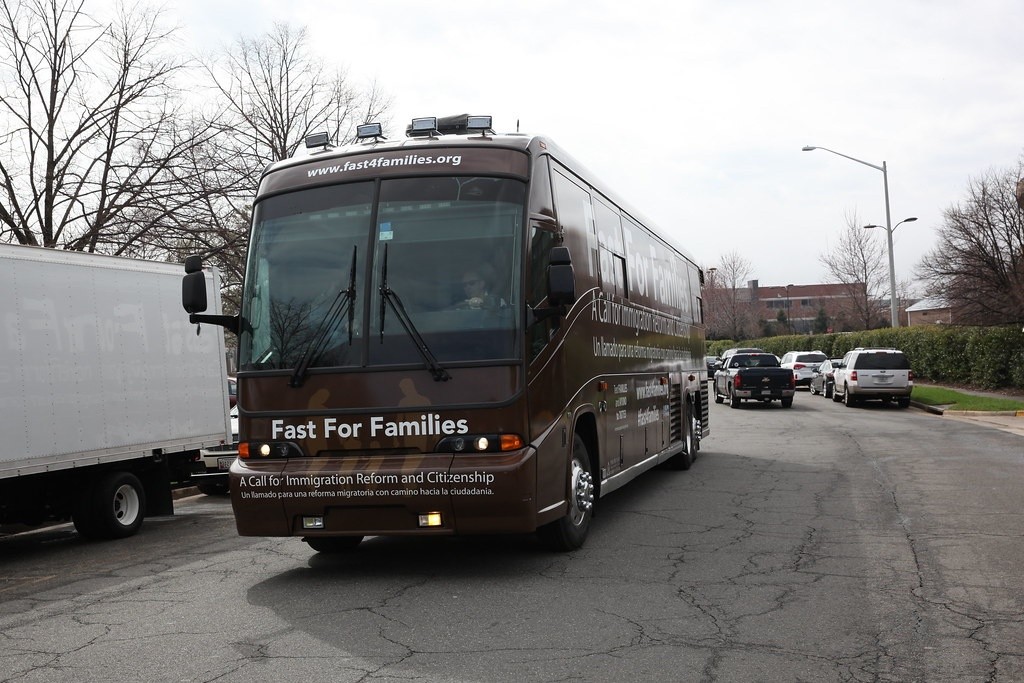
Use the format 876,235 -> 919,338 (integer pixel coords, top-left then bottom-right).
180,113 -> 712,553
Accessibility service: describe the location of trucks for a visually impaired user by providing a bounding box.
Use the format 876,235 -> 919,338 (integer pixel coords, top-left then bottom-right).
0,241 -> 232,545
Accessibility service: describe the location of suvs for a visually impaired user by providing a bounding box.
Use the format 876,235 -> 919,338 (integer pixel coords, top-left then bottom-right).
832,346 -> 914,410
777,349 -> 828,389
712,347 -> 763,373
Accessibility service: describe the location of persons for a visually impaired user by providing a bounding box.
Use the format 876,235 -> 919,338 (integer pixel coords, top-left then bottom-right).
460,270 -> 506,310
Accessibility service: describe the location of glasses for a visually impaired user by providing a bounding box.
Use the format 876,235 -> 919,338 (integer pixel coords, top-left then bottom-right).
464,280 -> 481,287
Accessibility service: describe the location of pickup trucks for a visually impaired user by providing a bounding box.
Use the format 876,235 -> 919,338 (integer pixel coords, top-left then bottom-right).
713,352 -> 797,407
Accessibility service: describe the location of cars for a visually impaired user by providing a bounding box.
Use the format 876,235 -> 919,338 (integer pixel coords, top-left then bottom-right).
810,358 -> 843,398
189,403 -> 241,496
227,377 -> 239,408
704,356 -> 721,377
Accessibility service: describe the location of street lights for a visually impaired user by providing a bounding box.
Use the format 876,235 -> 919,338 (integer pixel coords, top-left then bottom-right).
802,144 -> 918,329
769,284 -> 794,334
